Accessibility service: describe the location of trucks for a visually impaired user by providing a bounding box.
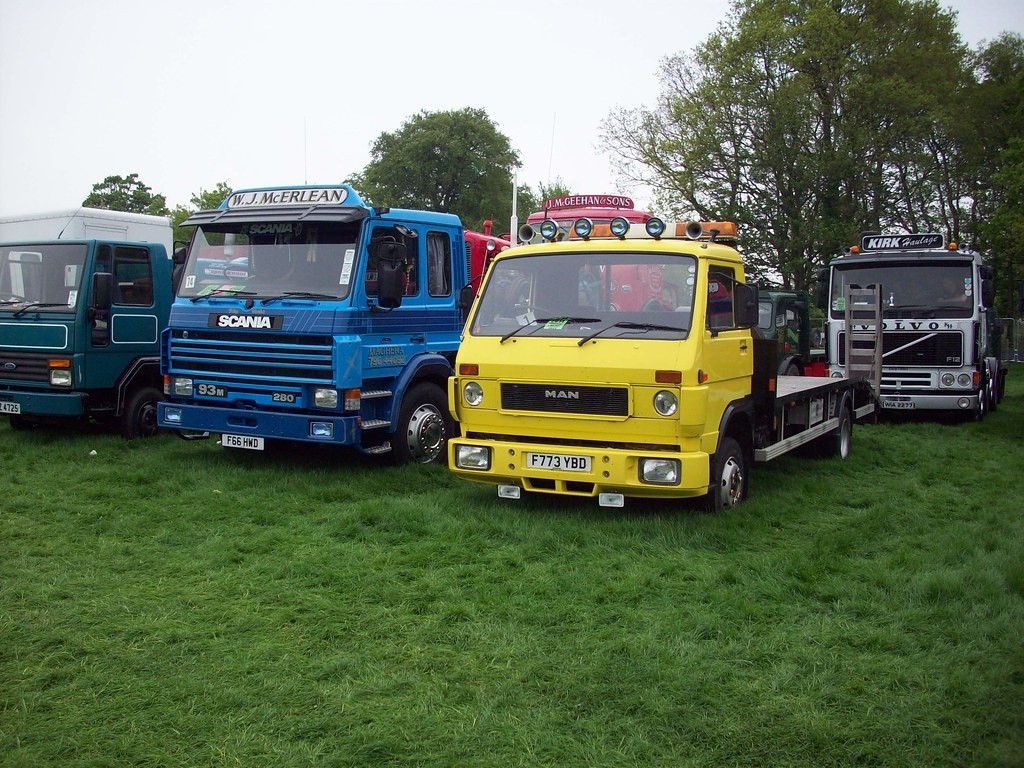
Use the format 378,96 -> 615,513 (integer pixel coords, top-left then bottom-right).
817,231 -> 1009,422
1,236 -> 190,441
155,183 -> 537,468
447,193 -> 883,514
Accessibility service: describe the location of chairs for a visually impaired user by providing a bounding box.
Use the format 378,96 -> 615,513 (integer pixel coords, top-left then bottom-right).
118,278 -> 151,305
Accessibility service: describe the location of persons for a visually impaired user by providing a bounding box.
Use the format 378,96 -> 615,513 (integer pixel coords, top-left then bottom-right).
364,234 -> 417,297
